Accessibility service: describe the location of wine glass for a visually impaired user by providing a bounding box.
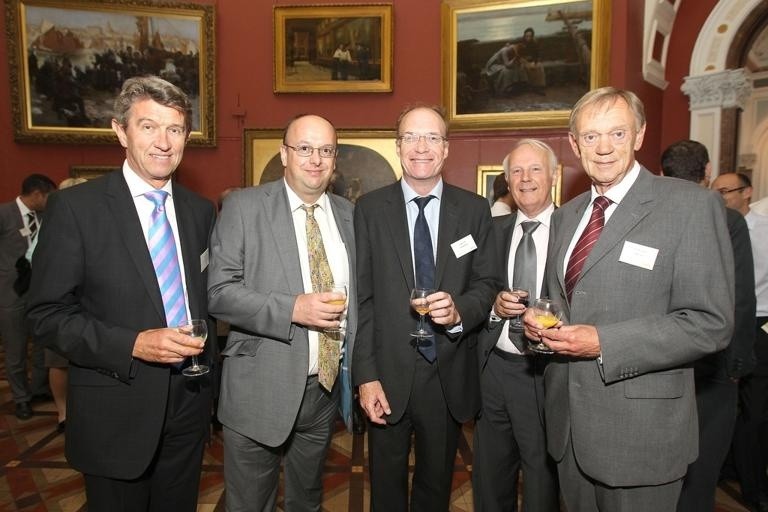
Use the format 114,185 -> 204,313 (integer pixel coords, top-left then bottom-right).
506,287 -> 532,331
528,300 -> 560,355
320,284 -> 347,334
409,288 -> 438,338
176,319 -> 212,376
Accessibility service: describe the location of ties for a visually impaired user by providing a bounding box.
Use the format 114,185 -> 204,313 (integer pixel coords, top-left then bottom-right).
564,196 -> 612,306
413,195 -> 437,364
508,222 -> 540,352
301,203 -> 340,393
143,189 -> 189,370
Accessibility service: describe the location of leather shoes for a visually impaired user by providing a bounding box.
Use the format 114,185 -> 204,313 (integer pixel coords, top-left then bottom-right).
15,390 -> 54,419
57,419 -> 66,433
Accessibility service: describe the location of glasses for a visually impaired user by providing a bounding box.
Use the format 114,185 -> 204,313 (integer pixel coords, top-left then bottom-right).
396,133 -> 448,145
719,185 -> 748,196
283,142 -> 337,159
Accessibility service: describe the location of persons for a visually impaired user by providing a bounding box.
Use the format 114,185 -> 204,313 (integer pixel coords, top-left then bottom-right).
13,176 -> 89,432
332,41 -> 371,81
207,112 -> 356,512
1,173 -> 58,414
20,73 -> 221,512
518,84 -> 736,512
710,172 -> 768,512
28,43 -> 200,128
517,28 -> 547,97
658,139 -> 756,512
490,172 -> 519,218
485,37 -> 529,96
469,139 -> 565,512
352,102 -> 504,511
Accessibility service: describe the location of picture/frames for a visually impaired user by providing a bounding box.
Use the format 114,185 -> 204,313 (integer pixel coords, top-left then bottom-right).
71,166 -> 122,181
4,0 -> 218,149
476,164 -> 562,210
244,128 -> 403,204
439,0 -> 612,132
272,2 -> 395,94
